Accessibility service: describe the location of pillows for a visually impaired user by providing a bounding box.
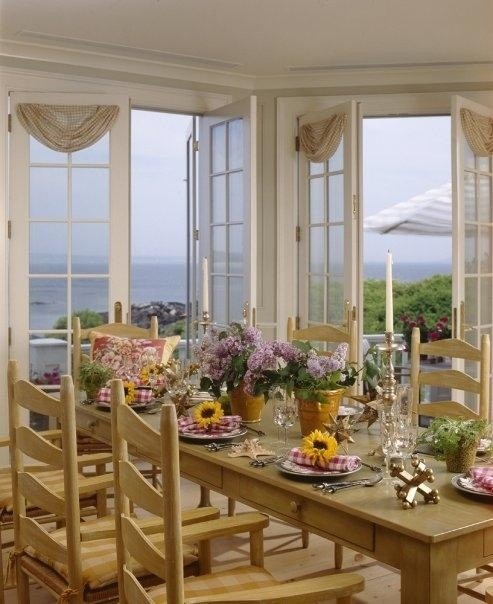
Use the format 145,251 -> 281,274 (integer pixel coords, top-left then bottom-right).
87,328 -> 179,372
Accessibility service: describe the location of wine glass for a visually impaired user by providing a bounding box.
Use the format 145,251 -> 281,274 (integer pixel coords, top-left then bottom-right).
376,383 -> 418,481
272,399 -> 296,453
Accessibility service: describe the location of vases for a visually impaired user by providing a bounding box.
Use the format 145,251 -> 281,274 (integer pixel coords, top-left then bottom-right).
225,385 -> 267,421
292,384 -> 343,437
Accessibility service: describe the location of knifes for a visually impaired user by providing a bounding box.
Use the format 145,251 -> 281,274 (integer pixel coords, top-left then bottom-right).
312,479 -> 372,489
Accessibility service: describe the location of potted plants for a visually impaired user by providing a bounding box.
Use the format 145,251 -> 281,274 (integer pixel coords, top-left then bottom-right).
77,361 -> 109,398
427,412 -> 486,473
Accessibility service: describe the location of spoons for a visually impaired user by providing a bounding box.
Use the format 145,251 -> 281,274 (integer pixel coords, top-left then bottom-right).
322,477 -> 384,493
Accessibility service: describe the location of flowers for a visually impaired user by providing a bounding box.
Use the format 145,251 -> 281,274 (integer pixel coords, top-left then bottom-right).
194,322 -> 260,396
194,401 -> 224,428
401,313 -> 429,340
302,429 -> 337,466
243,336 -> 390,399
428,316 -> 454,339
120,379 -> 133,403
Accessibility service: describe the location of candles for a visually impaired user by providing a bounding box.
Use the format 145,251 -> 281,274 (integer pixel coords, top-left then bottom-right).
385,249 -> 393,332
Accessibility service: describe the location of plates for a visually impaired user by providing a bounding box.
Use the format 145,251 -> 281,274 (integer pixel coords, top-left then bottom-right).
177,417 -> 248,439
94,397 -> 148,409
273,455 -> 362,477
188,390 -> 213,406
338,404 -> 363,415
451,474 -> 493,496
425,434 -> 492,453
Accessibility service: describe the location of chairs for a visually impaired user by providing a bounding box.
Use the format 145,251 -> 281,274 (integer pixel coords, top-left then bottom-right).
284,316 -> 358,406
106,376 -> 366,604
403,325 -> 490,438
71,310 -> 164,389
0,426 -> 108,524
10,371 -> 198,602
